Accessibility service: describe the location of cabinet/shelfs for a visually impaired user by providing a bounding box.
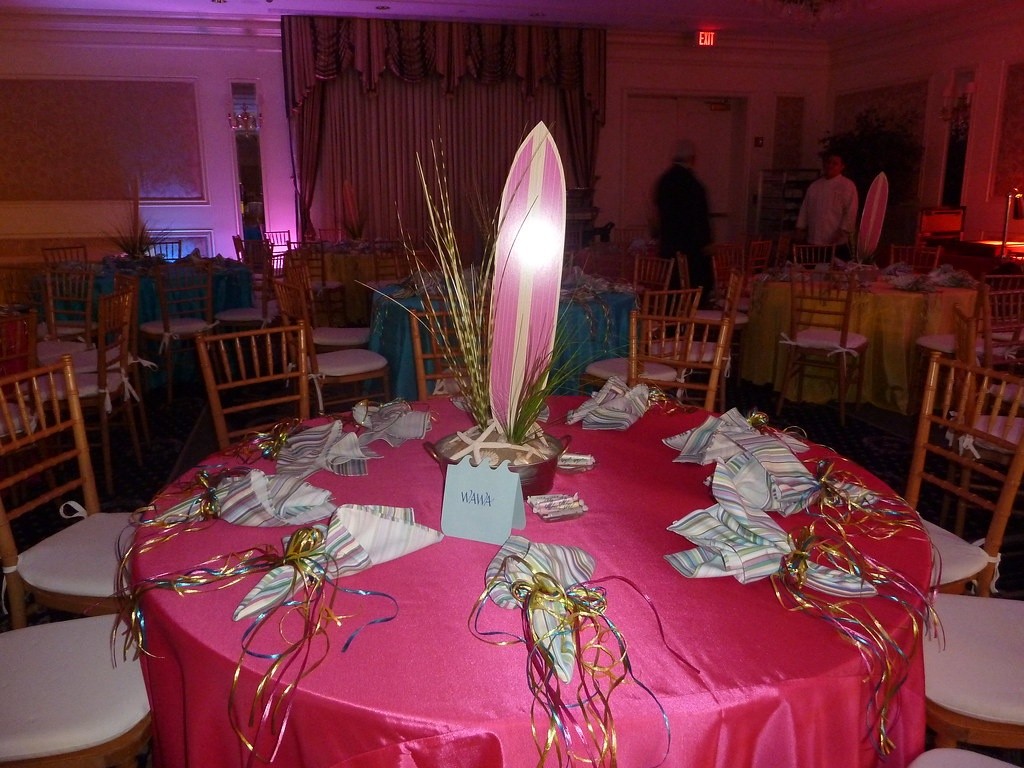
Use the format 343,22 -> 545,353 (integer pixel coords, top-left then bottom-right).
754,166 -> 820,266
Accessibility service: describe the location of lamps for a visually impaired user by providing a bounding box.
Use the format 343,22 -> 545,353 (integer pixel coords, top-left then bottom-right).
227,103 -> 263,139
996,185 -> 1024,261
938,80 -> 977,140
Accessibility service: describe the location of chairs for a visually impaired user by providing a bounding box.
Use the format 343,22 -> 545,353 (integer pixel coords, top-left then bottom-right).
937,302 -> 1023,599
268,274 -> 392,419
0,354 -> 144,630
906,349 -> 1023,598
41,269 -> 151,450
0,307 -> 38,507
137,259 -> 214,403
422,291 -> 490,373
194,317 -> 309,450
409,308 -> 492,401
0,227 -> 436,364
11,285 -> 145,497
921,592 -> 1024,750
0,612 -> 154,768
562,224 -> 1024,424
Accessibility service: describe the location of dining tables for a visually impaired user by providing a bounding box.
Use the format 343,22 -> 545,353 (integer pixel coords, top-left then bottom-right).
56,252 -> 253,400
0,303 -> 39,372
742,261 -> 980,417
360,285 -> 640,401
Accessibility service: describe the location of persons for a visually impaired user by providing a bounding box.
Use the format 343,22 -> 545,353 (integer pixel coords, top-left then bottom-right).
792,146 -> 858,263
652,139 -> 715,310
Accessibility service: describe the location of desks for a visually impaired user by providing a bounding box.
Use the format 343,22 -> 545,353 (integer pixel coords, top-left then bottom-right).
941,241 -> 1024,282
133,394 -> 933,767
